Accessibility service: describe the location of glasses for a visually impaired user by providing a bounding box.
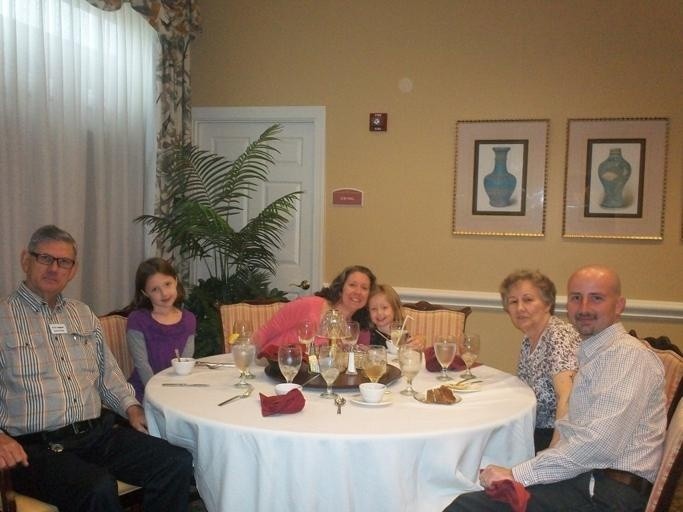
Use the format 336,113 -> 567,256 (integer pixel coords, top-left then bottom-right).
29,251 -> 75,269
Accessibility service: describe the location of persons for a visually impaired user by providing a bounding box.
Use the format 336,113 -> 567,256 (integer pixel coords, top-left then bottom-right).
443,266 -> 668,512
499,269 -> 583,452
0,224 -> 193,512
126,258 -> 197,404
367,285 -> 424,350
252,265 -> 376,353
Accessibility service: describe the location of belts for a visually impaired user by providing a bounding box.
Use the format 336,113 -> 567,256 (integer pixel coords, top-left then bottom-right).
607,469 -> 652,494
52,417 -> 98,436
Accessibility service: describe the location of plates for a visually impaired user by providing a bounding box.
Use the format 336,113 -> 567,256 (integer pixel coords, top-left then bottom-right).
346,397 -> 399,409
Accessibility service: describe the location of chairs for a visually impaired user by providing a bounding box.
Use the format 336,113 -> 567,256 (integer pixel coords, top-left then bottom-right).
99,314 -> 135,381
403,299 -> 472,348
645,383 -> 683,512
626,330 -> 683,416
0,479 -> 142,512
208,298 -> 291,354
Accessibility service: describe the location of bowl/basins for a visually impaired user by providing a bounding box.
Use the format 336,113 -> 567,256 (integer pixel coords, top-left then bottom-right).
169,357 -> 196,376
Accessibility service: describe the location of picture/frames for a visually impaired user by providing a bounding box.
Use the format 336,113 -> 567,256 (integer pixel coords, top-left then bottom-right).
561,116 -> 671,242
451,118 -> 551,237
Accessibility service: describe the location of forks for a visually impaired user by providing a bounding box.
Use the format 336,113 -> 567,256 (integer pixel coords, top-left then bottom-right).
217,387 -> 254,406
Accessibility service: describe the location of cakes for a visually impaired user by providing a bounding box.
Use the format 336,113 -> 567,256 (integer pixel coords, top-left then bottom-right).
427,385 -> 456,403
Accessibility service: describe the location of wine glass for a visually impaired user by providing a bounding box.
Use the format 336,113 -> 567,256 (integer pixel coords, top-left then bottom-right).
230,319 -> 257,388
276,308 -> 481,398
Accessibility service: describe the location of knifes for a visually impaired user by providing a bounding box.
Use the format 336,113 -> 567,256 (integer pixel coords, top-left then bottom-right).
161,382 -> 210,388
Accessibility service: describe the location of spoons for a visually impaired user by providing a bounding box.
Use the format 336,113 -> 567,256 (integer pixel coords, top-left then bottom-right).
334,399 -> 347,415
